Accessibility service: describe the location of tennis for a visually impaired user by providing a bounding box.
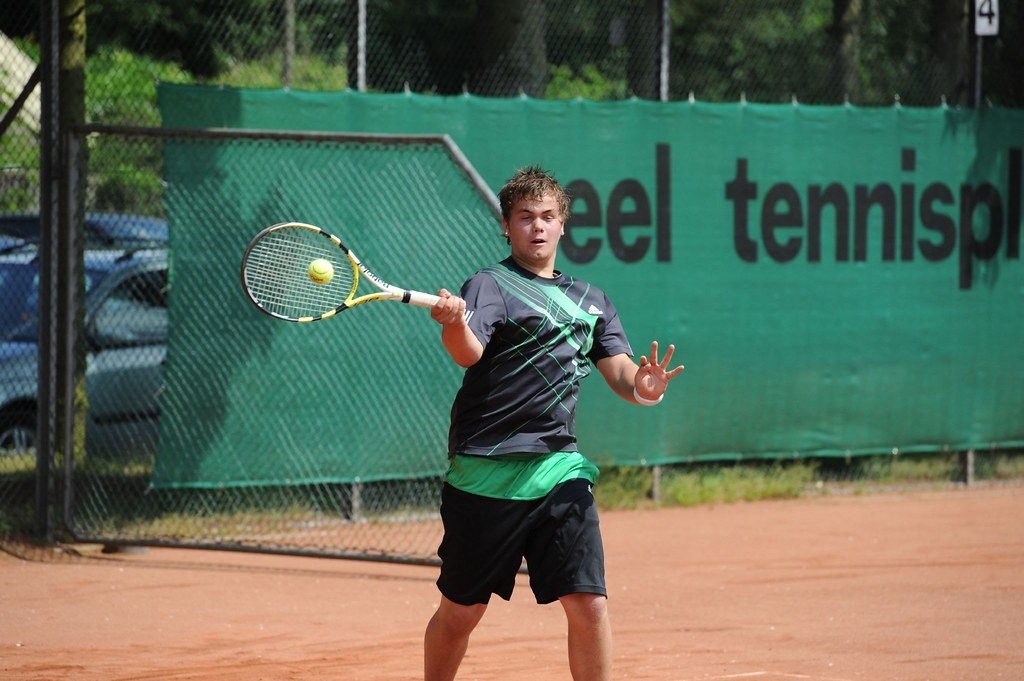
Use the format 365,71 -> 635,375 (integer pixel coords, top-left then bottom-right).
308,258 -> 335,284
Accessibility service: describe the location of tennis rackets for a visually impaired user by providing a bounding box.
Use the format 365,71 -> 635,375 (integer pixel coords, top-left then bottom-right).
240,221 -> 440,324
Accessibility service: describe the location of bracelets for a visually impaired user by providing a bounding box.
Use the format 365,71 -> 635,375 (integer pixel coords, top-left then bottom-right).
633,386 -> 664,407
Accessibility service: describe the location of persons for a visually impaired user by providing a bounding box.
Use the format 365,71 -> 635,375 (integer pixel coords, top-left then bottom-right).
424,167 -> 685,680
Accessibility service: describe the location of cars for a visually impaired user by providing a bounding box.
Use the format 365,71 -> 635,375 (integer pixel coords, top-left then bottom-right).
0,209 -> 171,251
0,248 -> 169,459
